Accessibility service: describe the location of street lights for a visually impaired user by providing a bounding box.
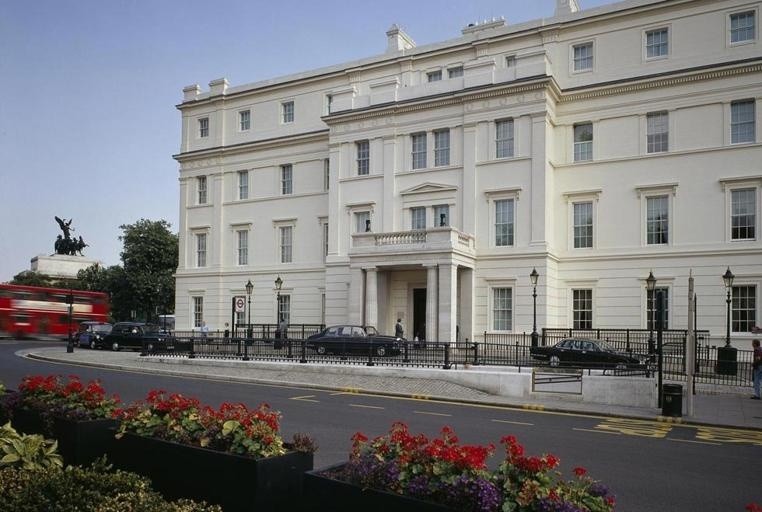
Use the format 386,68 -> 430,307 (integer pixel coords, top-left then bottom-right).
275,273 -> 283,349
529,265 -> 540,347
245,279 -> 254,346
722,265 -> 735,347
645,270 -> 658,362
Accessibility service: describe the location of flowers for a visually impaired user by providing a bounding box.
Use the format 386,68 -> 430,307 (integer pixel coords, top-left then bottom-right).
12,377 -> 286,454
350,422 -> 616,511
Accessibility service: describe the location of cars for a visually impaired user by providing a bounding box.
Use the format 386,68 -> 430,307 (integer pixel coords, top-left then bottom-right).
529,338 -> 640,369
78,321 -> 176,351
308,325 -> 408,356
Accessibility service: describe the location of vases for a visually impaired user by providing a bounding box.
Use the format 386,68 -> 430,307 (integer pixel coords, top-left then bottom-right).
107,425 -> 312,507
305,458 -> 431,510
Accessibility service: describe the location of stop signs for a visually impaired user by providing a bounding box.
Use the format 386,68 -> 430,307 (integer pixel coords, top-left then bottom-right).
236,297 -> 246,313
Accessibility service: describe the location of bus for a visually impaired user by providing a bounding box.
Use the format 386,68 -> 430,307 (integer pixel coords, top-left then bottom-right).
1,284 -> 110,340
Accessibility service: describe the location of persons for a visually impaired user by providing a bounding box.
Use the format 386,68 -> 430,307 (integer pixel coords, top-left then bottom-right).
395,318 -> 404,337
749,339 -> 762,399
223,322 -> 230,344
200,321 -> 209,348
276,319 -> 289,340
416,323 -> 425,348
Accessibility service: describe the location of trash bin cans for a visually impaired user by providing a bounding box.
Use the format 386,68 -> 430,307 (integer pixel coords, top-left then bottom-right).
718,347 -> 737,376
662,384 -> 682,417
